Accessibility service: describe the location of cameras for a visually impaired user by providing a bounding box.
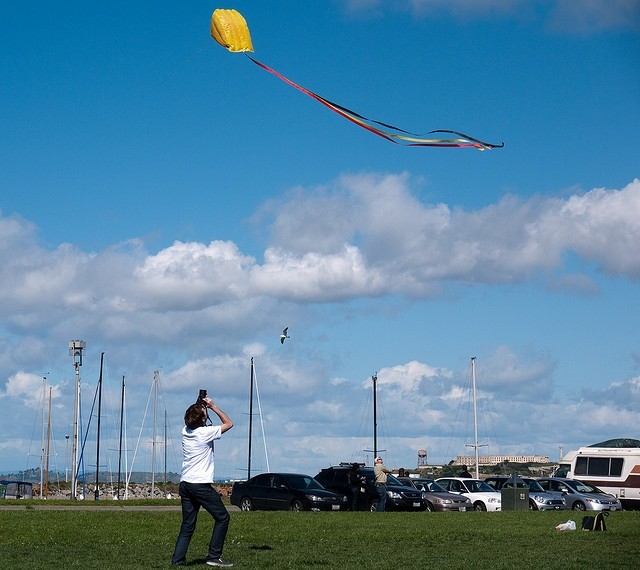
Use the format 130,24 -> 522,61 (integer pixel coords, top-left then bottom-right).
196,389 -> 208,406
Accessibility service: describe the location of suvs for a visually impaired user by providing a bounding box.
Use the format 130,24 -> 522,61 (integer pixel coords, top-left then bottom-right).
313,462 -> 428,512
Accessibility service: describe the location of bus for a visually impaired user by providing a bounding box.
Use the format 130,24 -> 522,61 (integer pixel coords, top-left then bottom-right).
550,446 -> 640,510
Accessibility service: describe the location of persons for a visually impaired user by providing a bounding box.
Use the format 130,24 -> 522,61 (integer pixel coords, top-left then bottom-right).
373,456 -> 396,512
171,394 -> 236,568
459,465 -> 472,478
349,462 -> 366,512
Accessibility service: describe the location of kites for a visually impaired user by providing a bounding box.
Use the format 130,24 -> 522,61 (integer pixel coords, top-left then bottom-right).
211,8 -> 505,151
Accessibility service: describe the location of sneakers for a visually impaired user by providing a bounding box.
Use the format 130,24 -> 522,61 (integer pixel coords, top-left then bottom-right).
206,556 -> 233,567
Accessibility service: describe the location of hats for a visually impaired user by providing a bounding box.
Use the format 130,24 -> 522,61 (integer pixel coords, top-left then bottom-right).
374,455 -> 381,463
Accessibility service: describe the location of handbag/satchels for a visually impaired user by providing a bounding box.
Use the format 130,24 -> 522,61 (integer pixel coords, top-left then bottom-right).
581,512 -> 609,531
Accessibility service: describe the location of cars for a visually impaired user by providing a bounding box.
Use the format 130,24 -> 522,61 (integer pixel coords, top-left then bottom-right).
530,478 -> 623,511
480,476 -> 567,511
421,477 -> 501,511
397,476 -> 474,512
230,473 -> 348,512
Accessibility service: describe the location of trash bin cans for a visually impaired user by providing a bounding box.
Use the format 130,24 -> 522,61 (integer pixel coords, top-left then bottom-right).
501,473 -> 530,511
